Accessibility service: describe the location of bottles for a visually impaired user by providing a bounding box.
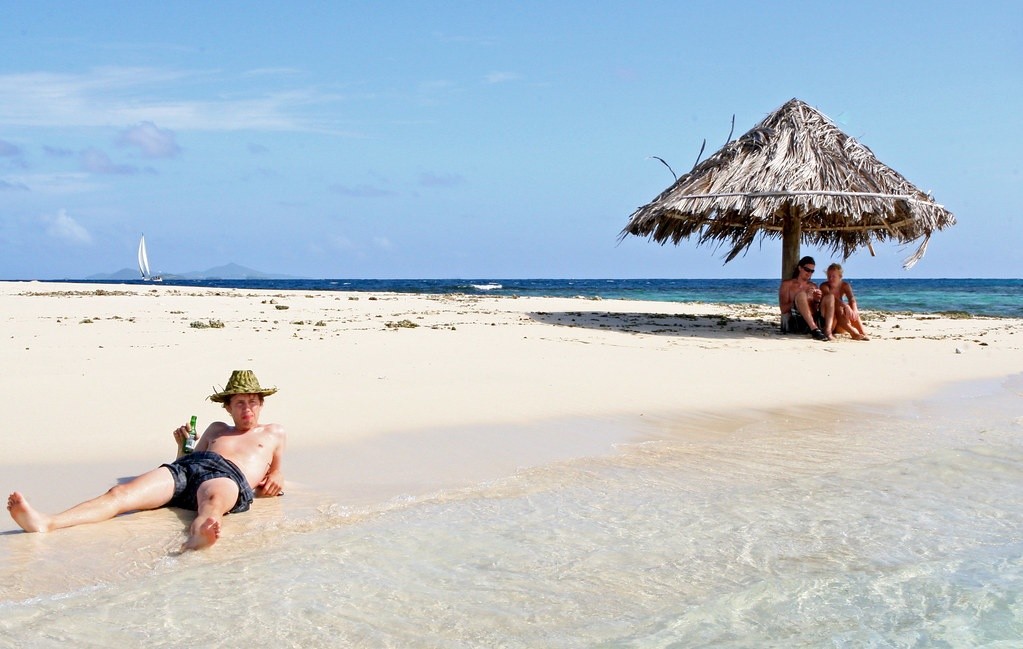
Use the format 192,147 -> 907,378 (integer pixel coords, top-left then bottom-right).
182,416 -> 199,453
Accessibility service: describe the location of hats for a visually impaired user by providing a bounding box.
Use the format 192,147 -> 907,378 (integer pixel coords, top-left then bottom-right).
209,369 -> 277,403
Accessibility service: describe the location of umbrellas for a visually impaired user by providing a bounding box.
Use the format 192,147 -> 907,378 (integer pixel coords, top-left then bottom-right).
625,98 -> 956,280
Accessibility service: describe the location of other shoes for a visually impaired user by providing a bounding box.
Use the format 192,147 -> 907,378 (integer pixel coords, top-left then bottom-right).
853,334 -> 870,341
811,328 -> 825,340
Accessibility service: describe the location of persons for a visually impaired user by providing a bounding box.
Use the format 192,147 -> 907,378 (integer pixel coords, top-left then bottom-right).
7,370 -> 288,554
779,256 -> 836,341
819,263 -> 870,341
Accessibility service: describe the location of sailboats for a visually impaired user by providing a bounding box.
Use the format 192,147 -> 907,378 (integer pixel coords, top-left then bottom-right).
138,232 -> 162,282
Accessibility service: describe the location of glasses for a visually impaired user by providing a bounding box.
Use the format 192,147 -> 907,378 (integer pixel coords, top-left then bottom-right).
802,267 -> 815,273
